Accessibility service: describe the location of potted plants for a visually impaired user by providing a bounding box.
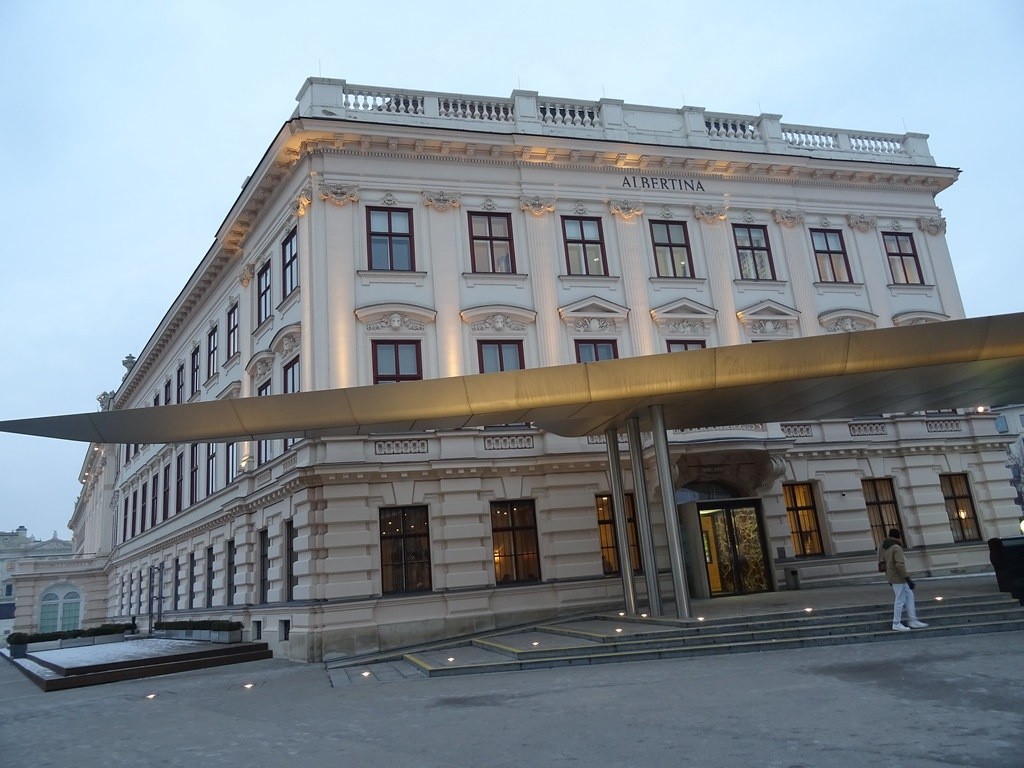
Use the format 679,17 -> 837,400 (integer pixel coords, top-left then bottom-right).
7,623 -> 139,659
154,620 -> 244,644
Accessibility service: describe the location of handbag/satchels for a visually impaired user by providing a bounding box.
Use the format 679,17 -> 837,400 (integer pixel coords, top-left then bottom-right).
878,561 -> 886,572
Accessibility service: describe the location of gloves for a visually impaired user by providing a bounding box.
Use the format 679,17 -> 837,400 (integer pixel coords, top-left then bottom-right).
906,579 -> 915,590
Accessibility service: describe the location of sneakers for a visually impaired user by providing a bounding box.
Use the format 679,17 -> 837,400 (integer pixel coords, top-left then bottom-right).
907,620 -> 928,628
892,623 -> 910,631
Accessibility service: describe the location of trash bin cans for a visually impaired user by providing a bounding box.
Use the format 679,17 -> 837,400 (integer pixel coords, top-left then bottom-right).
783,567 -> 801,590
988,535 -> 1024,606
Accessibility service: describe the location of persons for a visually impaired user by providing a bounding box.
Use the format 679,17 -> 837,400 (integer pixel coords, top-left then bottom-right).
879,528 -> 929,631
600,555 -> 613,573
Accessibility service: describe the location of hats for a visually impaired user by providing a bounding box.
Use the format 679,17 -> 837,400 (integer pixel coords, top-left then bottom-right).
888,529 -> 901,538
